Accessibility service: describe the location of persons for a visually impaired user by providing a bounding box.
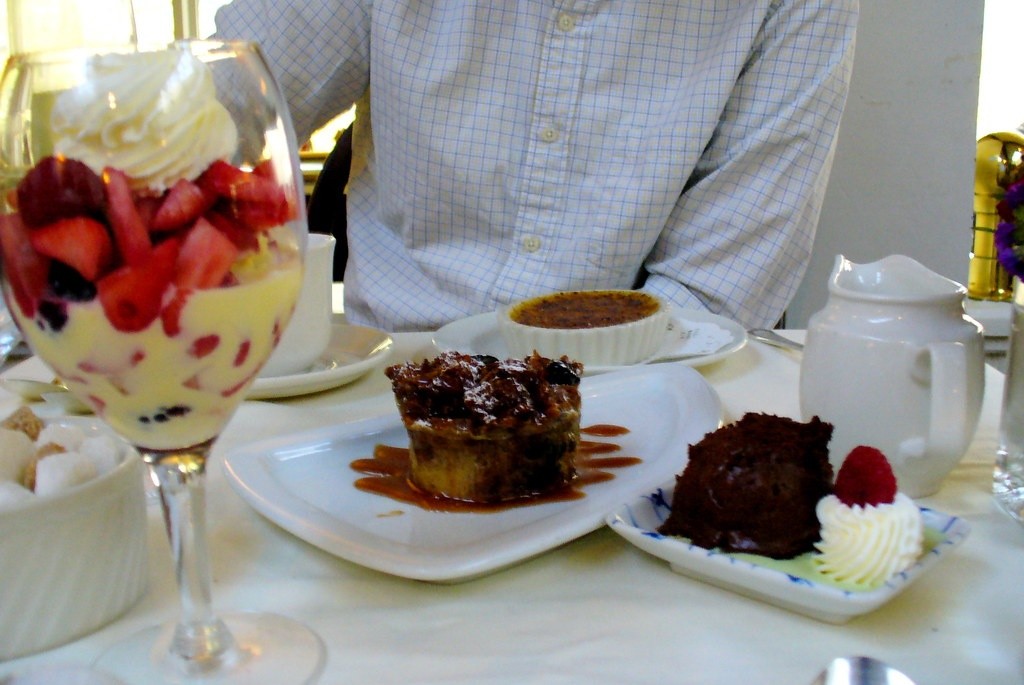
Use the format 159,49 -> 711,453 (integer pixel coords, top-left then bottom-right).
198,0 -> 861,335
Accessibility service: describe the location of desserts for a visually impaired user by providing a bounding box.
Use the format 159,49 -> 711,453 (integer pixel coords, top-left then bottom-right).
654,411 -> 922,591
382,348 -> 581,505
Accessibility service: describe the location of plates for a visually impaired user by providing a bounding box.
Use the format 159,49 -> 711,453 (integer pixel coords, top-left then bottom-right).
220,364 -> 723,584
431,310 -> 749,365
607,481 -> 973,625
243,325 -> 394,399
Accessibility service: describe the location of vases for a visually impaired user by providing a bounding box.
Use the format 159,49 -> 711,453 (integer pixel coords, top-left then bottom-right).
986,304 -> 1024,521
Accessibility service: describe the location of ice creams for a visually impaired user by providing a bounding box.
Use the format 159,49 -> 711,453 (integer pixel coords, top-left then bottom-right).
51,46 -> 240,193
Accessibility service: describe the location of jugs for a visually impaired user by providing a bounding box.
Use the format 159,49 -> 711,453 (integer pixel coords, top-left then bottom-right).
800,253 -> 985,498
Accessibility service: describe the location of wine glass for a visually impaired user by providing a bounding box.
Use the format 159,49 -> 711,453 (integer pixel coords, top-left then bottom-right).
0,40 -> 327,682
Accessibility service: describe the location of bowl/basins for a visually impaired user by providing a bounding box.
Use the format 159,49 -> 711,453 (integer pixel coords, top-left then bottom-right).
504,289 -> 669,367
0,438 -> 147,662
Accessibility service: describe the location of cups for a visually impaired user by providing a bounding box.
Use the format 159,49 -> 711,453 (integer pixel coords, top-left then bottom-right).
992,276 -> 1024,522
248,233 -> 336,379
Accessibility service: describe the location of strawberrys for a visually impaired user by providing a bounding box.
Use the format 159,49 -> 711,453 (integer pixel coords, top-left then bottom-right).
1,156 -> 294,332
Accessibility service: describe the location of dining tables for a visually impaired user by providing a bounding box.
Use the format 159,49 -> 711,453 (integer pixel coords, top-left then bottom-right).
1,322 -> 1024,685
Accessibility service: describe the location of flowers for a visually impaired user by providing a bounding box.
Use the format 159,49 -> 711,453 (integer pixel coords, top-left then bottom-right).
991,155 -> 1024,293
